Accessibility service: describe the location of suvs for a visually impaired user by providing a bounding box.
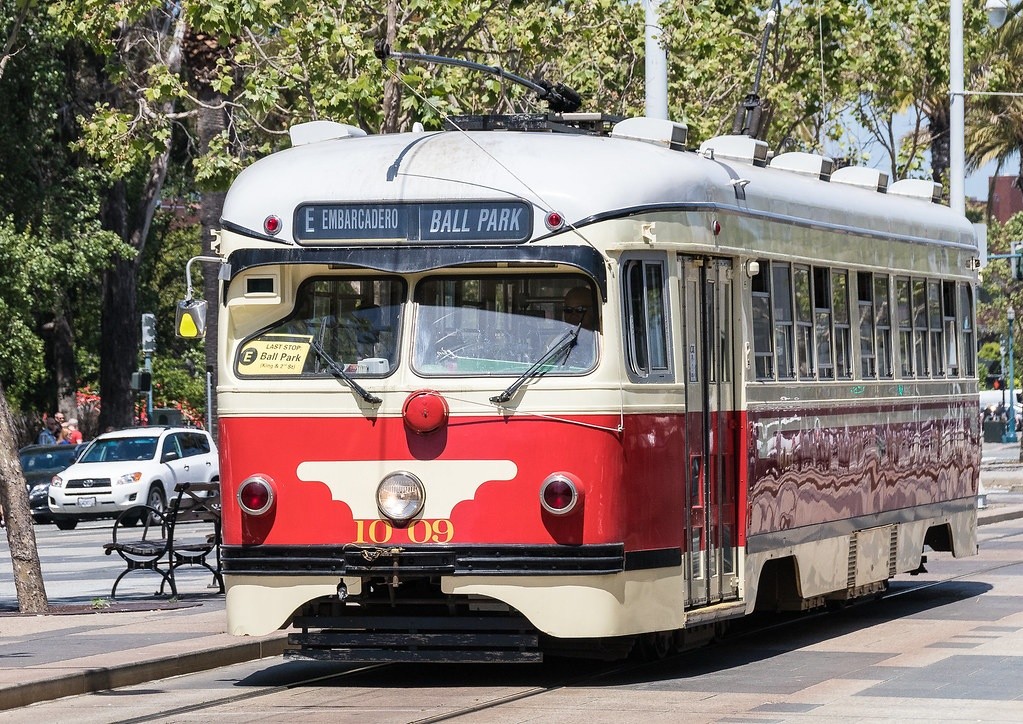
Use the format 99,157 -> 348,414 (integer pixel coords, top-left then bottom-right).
49,429 -> 220,529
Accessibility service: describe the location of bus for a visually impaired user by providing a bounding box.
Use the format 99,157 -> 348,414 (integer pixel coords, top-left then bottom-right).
174,113 -> 983,668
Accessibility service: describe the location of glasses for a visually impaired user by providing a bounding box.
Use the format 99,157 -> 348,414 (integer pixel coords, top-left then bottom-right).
562,305 -> 592,314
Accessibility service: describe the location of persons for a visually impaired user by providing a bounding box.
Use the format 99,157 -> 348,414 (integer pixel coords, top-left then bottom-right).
536,286 -> 596,368
37,412 -> 82,446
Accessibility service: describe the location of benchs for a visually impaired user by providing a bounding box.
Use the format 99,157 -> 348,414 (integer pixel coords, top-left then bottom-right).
102,482 -> 225,599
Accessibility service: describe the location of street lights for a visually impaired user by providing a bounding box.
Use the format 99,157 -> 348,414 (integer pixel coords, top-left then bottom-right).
1007,306 -> 1017,441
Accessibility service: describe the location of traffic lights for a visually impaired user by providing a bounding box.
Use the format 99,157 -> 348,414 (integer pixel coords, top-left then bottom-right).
985,376 -> 1004,390
132,372 -> 151,391
1016,392 -> 1023,404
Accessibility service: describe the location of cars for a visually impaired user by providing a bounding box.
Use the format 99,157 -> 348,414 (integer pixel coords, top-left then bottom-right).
0,443 -> 119,524
980,403 -> 1023,431
